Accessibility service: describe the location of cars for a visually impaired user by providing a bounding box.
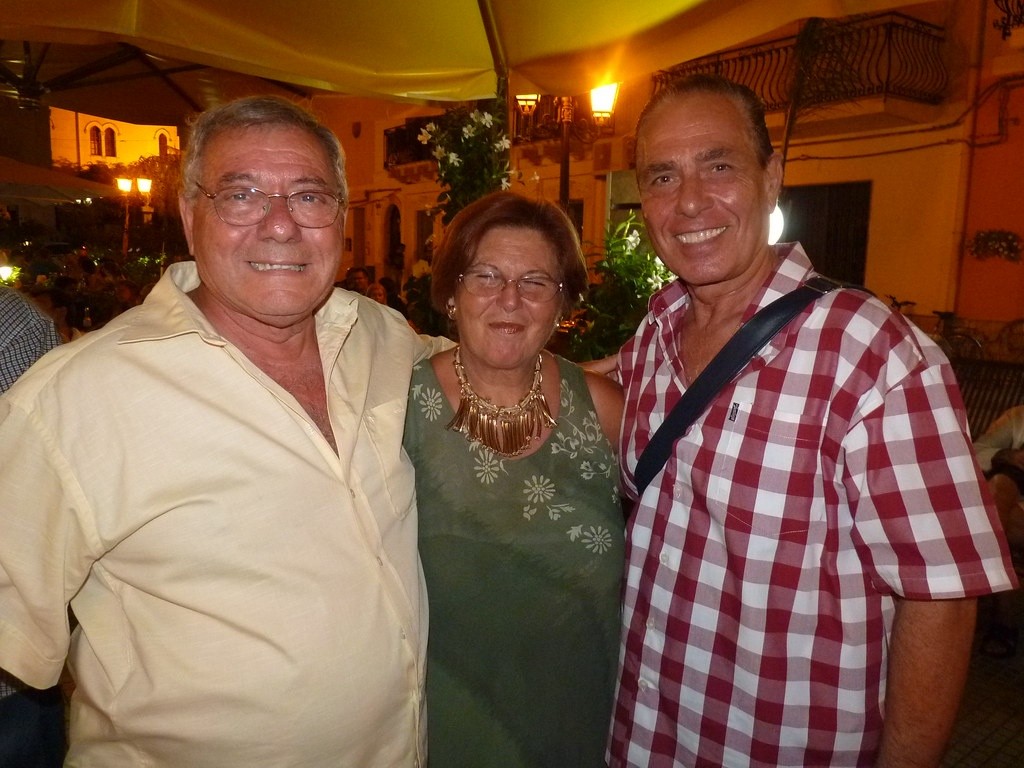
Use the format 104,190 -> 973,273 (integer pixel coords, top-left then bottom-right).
17,241 -> 86,280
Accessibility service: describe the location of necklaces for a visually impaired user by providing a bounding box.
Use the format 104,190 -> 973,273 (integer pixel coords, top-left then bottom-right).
444,345 -> 558,459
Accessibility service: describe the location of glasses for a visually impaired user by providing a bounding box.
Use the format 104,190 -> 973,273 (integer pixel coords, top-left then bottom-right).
191,179 -> 345,228
458,262 -> 568,302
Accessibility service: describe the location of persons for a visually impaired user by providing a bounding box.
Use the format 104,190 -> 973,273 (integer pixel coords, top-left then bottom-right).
970,404 -> 1024,659
342,266 -> 409,321
0,96 -> 619,768
11,250 -> 155,344
0,282 -> 58,394
602,74 -> 1024,768
403,192 -> 628,768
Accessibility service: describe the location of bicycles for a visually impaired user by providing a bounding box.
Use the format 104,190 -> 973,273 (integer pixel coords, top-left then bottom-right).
889,294 -> 982,376
